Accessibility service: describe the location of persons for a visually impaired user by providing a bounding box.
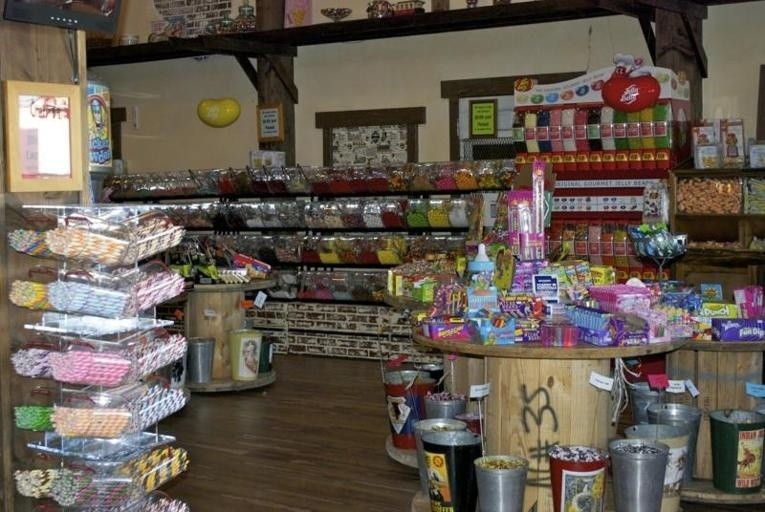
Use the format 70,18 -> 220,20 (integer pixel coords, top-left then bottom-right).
242,340 -> 258,374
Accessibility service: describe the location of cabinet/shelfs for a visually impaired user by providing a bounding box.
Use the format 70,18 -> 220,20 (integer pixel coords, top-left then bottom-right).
667,167 -> 764,304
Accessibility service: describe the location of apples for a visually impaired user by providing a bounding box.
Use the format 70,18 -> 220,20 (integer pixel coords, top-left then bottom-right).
600,74 -> 660,112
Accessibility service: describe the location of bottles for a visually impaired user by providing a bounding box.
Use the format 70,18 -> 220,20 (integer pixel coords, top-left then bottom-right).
474,242 -> 488,263
118,32 -> 138,46
205,1 -> 257,34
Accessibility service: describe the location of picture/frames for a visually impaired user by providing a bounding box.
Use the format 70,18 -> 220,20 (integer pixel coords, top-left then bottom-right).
256,101 -> 285,143
469,98 -> 498,139
2,76 -> 87,194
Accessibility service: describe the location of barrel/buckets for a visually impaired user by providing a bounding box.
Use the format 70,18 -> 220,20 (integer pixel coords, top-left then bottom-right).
228,330 -> 274,381
186,335 -> 216,384
548,441 -> 611,512
707,409 -> 765,495
605,382 -> 702,511
474,455 -> 529,511
380,361 -> 481,512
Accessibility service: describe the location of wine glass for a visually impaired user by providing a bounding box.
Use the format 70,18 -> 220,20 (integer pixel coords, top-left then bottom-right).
320,8 -> 351,24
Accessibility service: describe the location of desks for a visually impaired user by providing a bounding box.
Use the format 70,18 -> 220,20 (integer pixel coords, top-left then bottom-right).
660,339 -> 764,505
410,327 -> 689,512
180,277 -> 280,393
